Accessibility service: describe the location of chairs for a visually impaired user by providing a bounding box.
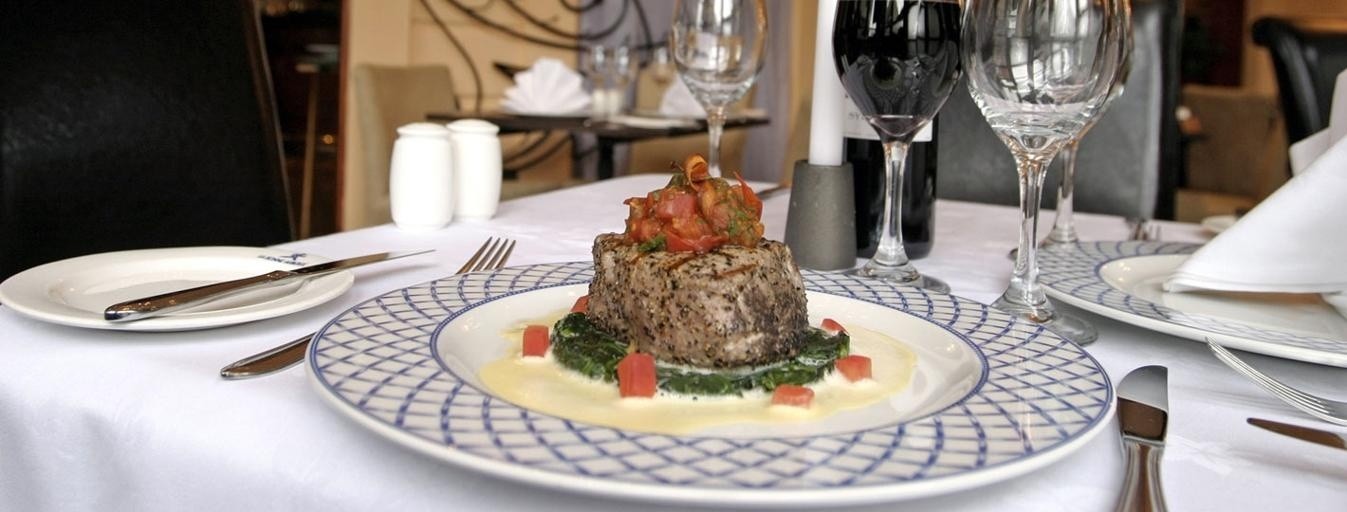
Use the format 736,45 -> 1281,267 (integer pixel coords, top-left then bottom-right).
614,65 -> 758,172
358,65 -> 557,221
1250,17 -> 1347,166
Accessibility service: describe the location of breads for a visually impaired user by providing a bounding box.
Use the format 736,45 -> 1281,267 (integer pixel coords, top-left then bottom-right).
477,151 -> 916,434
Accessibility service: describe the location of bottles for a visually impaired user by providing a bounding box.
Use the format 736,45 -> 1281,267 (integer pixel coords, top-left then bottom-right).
383,118 -> 503,227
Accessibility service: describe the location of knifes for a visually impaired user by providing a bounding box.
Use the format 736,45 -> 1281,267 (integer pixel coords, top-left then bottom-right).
1105,363 -> 1174,510
97,246 -> 435,321
1246,415 -> 1345,450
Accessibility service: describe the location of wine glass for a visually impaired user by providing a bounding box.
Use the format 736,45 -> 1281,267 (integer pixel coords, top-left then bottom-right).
833,1 -> 968,295
670,1 -> 770,200
959,1 -> 1138,346
581,39 -> 676,127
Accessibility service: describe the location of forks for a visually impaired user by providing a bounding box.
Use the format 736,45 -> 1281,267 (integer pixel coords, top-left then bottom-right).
221,237 -> 523,391
1200,341 -> 1345,427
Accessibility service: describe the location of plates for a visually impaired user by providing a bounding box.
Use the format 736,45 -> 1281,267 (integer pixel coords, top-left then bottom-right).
1012,239 -> 1346,369
309,259 -> 1116,503
1,247 -> 354,330
